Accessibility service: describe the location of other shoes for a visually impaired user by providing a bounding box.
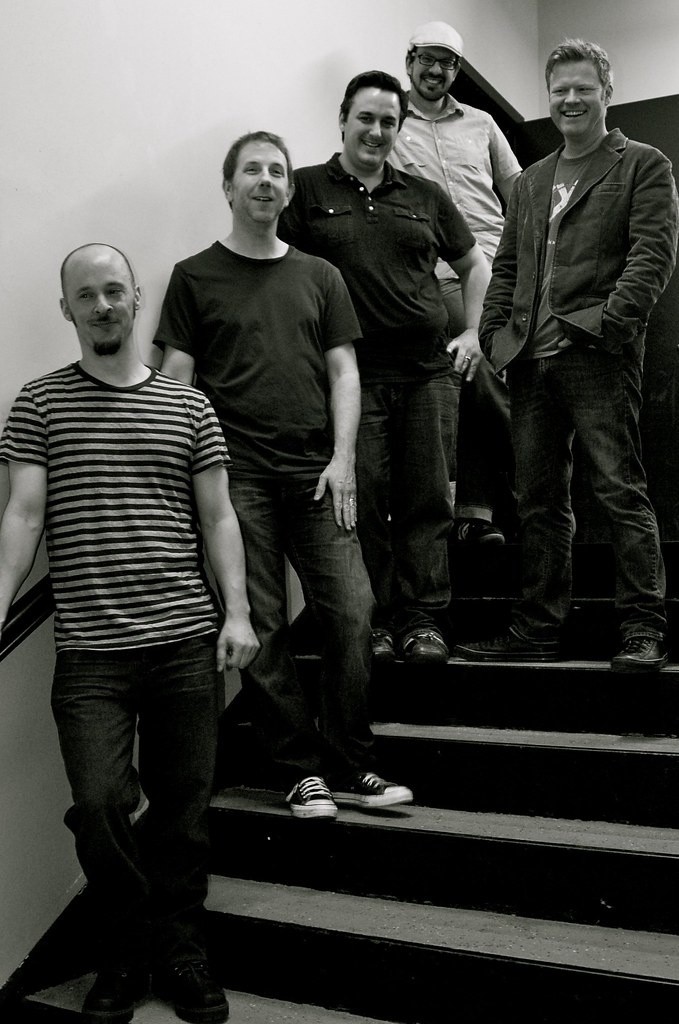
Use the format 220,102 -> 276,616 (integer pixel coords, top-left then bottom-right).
81,953 -> 151,1024
400,628 -> 449,664
153,955 -> 230,1024
373,631 -> 395,660
450,519 -> 505,546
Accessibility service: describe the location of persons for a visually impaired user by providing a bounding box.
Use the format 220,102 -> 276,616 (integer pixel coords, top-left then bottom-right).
0,240 -> 259,1024
277,68 -> 491,663
453,40 -> 679,668
384,21 -> 524,543
151,131 -> 414,820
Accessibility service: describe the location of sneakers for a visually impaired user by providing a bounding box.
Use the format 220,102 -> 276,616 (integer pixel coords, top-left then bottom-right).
285,775 -> 338,818
612,637 -> 669,672
329,772 -> 414,808
452,626 -> 557,661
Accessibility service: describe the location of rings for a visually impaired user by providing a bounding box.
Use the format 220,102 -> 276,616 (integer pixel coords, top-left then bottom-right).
464,356 -> 471,360
350,498 -> 356,501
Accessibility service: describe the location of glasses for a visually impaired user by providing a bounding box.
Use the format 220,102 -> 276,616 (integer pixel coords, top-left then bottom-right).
410,52 -> 458,70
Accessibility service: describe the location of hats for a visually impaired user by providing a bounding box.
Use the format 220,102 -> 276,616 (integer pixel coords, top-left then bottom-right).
408,21 -> 464,58
